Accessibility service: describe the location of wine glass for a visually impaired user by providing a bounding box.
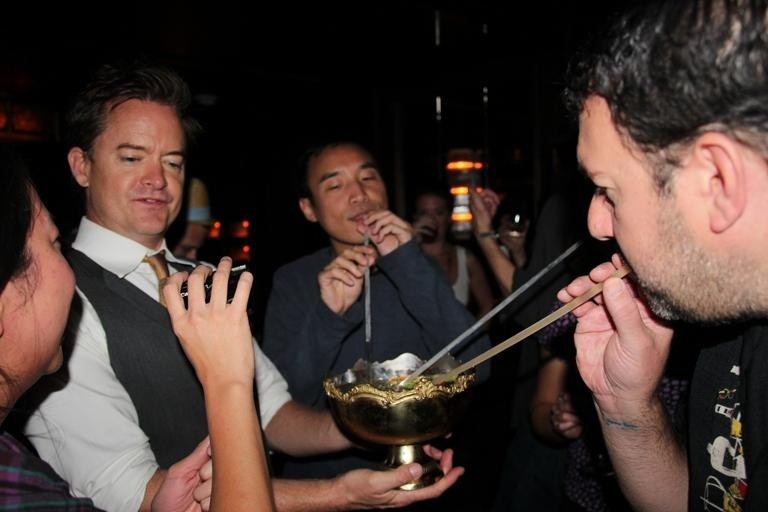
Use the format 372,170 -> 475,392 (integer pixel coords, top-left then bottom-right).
321,353 -> 476,492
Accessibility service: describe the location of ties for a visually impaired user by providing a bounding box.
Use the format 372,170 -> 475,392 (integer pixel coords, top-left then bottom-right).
143,249 -> 170,307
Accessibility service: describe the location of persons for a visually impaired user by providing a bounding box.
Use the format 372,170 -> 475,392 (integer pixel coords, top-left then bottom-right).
261,133 -> 494,477
0,151 -> 276,511
557,0 -> 768,512
471,173 -> 695,476
21,65 -> 465,512
406,186 -> 493,318
